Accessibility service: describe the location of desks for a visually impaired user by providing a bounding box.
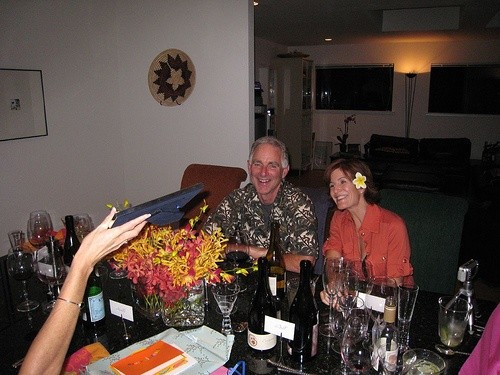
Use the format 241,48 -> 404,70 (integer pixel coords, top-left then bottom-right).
0,254 -> 498,375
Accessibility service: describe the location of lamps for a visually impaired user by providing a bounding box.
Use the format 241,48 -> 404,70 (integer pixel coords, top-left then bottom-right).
405,73 -> 417,137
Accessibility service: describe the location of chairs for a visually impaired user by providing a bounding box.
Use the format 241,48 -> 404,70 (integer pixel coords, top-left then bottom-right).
298,186 -> 330,275
180,164 -> 248,232
378,189 -> 467,294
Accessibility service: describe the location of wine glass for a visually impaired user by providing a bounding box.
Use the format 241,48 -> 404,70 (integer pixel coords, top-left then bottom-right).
8,211 -> 419,375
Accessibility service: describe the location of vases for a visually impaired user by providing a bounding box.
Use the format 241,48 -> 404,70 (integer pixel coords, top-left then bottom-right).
130,282 -> 205,326
340,144 -> 346,152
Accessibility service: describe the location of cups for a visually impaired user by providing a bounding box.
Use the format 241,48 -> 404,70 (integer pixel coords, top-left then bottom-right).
438,296 -> 473,348
402,348 -> 447,375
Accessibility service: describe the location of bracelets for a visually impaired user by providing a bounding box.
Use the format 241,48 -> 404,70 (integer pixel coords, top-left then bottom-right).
56,298 -> 81,308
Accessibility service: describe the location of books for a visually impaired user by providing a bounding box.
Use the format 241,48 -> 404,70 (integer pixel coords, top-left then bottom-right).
109,340 -> 200,375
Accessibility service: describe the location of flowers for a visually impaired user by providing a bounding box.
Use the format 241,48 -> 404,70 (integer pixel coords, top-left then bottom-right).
352,172 -> 367,190
335,113 -> 356,145
105,198 -> 258,308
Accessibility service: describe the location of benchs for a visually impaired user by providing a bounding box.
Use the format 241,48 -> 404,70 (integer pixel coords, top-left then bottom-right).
364,134 -> 471,194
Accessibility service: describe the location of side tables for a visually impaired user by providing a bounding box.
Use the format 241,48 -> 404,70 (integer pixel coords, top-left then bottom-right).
330,152 -> 361,163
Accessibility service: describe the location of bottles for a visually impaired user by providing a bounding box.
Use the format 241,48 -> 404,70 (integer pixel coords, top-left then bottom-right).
266,222 -> 287,297
53,246 -> 66,282
371,296 -> 401,373
46,239 -> 59,264
80,266 -> 107,339
248,256 -> 277,353
286,259 -> 319,361
62,215 -> 82,276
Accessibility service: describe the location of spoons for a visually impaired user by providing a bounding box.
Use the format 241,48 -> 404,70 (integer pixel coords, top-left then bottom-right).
434,344 -> 471,356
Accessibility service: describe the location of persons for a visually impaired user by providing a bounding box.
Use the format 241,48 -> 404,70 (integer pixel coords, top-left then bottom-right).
457,303 -> 500,375
18,206 -> 151,375
320,158 -> 414,308
201,136 -> 320,274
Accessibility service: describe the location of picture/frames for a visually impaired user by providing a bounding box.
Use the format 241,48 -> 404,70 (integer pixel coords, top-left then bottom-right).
0,68 -> 48,142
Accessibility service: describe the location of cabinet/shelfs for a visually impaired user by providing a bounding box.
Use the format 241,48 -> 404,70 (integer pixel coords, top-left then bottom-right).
276,57 -> 313,176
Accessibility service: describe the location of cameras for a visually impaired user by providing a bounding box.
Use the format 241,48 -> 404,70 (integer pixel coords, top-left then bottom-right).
457,260 -> 478,282
